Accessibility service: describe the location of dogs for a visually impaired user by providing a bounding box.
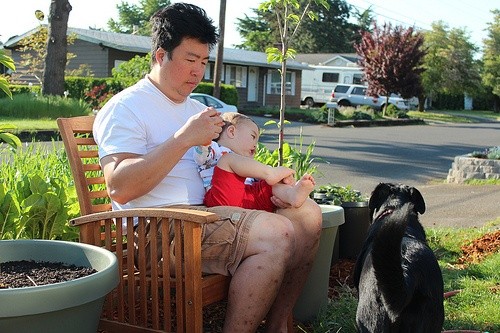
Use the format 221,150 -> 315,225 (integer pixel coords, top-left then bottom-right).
354,181 -> 445,333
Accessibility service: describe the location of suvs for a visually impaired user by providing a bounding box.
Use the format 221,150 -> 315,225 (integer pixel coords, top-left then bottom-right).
332,84 -> 409,115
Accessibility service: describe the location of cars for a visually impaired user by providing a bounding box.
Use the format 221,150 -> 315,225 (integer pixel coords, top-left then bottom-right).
189,93 -> 238,120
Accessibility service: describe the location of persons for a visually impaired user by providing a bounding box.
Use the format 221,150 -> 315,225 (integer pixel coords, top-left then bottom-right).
93,3 -> 322,332
193,107 -> 316,212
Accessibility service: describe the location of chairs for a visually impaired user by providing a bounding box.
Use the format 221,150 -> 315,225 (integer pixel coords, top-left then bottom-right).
57,114 -> 294,333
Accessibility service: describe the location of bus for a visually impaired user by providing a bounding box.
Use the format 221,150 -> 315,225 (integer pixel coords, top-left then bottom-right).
299,66 -> 398,108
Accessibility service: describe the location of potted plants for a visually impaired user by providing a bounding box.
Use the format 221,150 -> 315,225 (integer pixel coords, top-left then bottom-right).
314,183 -> 369,261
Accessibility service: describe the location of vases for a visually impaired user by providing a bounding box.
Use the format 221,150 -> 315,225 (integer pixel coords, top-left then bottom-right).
290,205 -> 346,323
0,240 -> 119,333
